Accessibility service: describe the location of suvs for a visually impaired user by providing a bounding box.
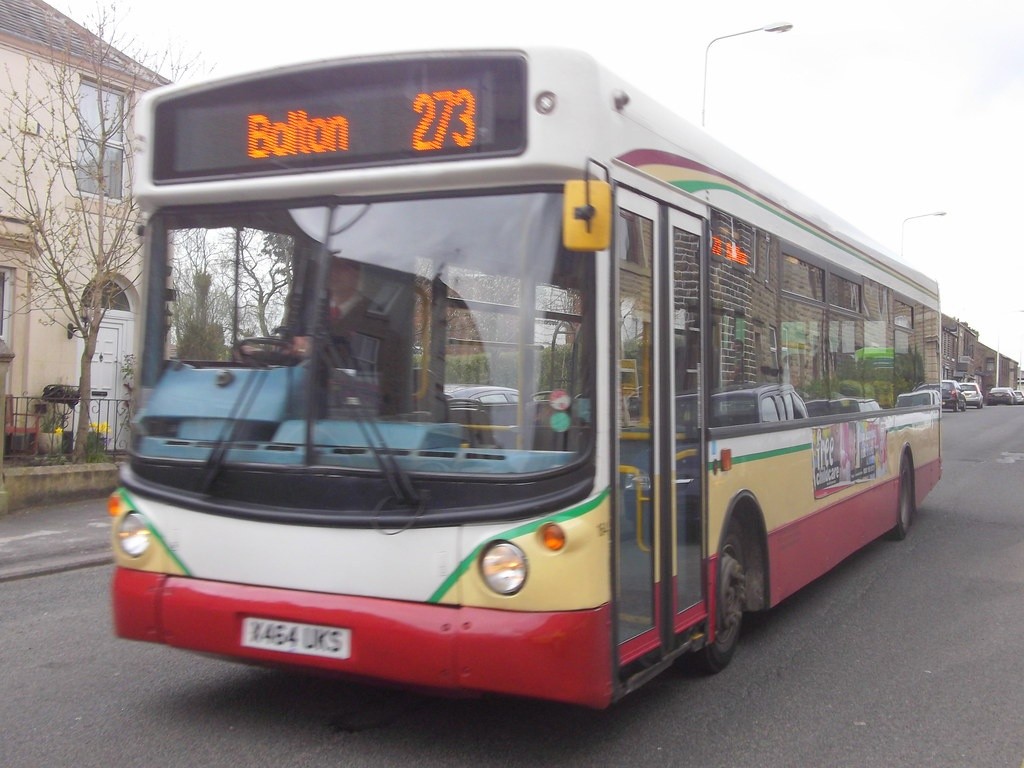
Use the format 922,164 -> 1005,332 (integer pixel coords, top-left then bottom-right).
442,383 -> 520,425
623,381 -> 811,522
958,383 -> 984,409
895,388 -> 941,408
943,379 -> 967,412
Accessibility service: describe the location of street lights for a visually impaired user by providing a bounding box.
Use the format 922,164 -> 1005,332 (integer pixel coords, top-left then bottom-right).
769,326 -> 780,382
994,308 -> 1024,388
782,328 -> 791,385
702,23 -> 795,129
901,212 -> 951,255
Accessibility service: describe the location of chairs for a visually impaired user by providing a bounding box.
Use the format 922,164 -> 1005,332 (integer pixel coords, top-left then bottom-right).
6,393 -> 39,456
448,399 -> 494,448
566,395 -> 582,451
522,390 -> 568,450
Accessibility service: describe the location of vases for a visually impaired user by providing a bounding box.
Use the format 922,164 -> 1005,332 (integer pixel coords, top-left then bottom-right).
39,432 -> 63,453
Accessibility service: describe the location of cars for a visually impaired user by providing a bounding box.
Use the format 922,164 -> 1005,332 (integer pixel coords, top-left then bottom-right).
987,387 -> 1024,406
805,397 -> 882,417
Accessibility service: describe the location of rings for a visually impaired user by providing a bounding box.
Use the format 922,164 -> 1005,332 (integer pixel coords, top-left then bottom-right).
298,348 -> 306,352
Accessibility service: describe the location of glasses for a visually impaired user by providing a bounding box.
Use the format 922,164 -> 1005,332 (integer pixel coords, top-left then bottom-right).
760,366 -> 780,377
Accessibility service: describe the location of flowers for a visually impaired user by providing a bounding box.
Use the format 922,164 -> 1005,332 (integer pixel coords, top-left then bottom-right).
39,414 -> 66,435
89,420 -> 113,433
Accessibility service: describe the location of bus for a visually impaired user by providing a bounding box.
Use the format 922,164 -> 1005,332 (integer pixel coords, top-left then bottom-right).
108,39 -> 945,715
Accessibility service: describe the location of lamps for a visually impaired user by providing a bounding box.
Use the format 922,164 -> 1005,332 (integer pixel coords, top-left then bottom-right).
68,302 -> 95,341
163,305 -> 174,343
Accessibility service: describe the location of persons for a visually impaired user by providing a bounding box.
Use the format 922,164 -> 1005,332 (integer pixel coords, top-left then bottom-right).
233,255 -> 409,416
